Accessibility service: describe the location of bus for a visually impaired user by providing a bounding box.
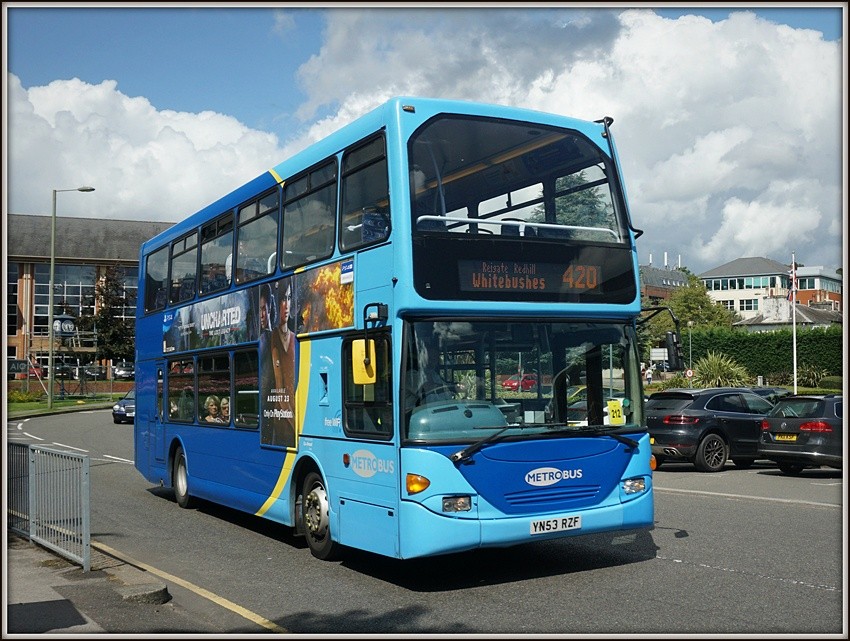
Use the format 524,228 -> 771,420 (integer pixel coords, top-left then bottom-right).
133,95 -> 685,563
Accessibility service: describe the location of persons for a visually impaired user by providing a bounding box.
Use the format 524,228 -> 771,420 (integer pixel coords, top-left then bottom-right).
259,276 -> 295,448
225,238 -> 253,285
645,367 -> 653,384
215,397 -> 229,424
202,395 -> 220,423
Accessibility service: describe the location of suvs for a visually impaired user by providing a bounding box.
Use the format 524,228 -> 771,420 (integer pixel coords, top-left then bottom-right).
758,393 -> 843,475
644,387 -> 785,472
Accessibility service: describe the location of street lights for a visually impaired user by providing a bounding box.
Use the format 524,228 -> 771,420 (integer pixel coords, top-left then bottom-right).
47,186 -> 96,409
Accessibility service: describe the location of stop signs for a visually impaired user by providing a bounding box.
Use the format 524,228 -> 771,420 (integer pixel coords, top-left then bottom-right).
641,369 -> 645,376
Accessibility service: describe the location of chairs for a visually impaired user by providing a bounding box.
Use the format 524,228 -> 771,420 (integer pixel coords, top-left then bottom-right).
155,207 -> 572,309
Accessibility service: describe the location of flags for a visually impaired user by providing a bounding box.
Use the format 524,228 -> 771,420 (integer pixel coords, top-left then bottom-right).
787,262 -> 798,301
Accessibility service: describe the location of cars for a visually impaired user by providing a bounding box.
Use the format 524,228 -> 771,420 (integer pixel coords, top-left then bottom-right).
655,362 -> 672,373
544,384 -> 649,428
501,373 -> 544,392
55,362 -> 135,381
26,363 -> 43,381
111,385 -> 136,425
